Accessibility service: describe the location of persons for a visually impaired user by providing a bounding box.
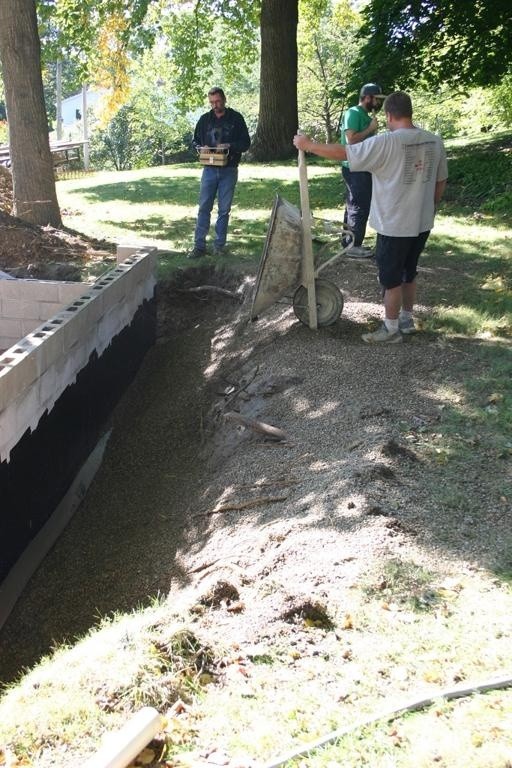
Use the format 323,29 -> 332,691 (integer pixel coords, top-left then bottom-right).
293,90 -> 449,345
186,86 -> 251,262
336,84 -> 384,263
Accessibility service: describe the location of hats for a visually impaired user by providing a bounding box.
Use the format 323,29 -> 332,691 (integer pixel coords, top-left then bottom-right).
359,82 -> 387,99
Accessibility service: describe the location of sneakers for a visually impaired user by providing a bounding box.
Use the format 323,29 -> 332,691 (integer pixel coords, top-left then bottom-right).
186,246 -> 225,259
360,317 -> 417,345
346,244 -> 373,257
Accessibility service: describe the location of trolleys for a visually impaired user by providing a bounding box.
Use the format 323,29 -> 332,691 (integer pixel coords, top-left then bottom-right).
245,128 -> 358,335
